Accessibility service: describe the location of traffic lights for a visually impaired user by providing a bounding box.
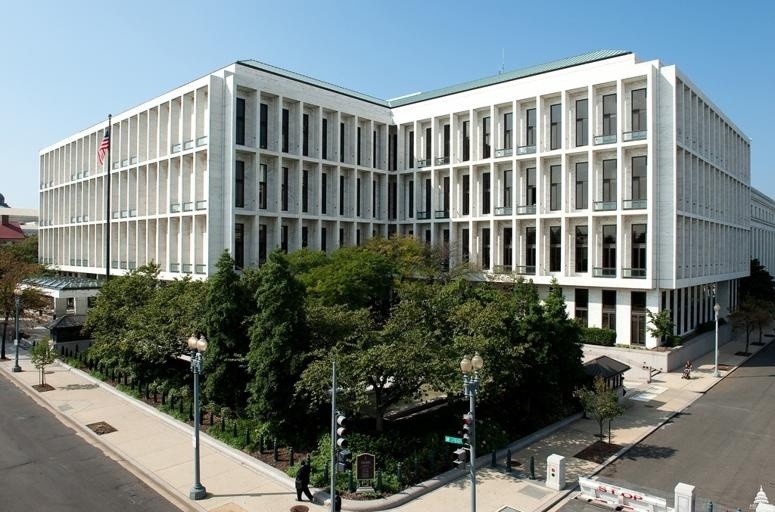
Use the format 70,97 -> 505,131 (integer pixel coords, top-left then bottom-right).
336,412 -> 347,454
454,448 -> 468,472
462,413 -> 473,447
337,451 -> 352,475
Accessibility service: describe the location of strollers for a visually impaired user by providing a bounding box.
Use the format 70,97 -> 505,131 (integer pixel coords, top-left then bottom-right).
682,369 -> 693,380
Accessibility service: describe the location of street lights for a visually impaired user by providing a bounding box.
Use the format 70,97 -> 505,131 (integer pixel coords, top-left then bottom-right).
461,352 -> 485,512
713,302 -> 722,378
11,288 -> 25,373
186,331 -> 210,502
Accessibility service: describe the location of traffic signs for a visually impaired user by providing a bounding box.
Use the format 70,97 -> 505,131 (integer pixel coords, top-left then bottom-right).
446,435 -> 465,447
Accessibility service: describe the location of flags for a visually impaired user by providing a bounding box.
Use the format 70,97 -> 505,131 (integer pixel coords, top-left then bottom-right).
96,128 -> 110,168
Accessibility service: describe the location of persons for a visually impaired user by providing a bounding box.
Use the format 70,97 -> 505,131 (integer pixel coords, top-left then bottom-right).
294,460 -> 315,503
